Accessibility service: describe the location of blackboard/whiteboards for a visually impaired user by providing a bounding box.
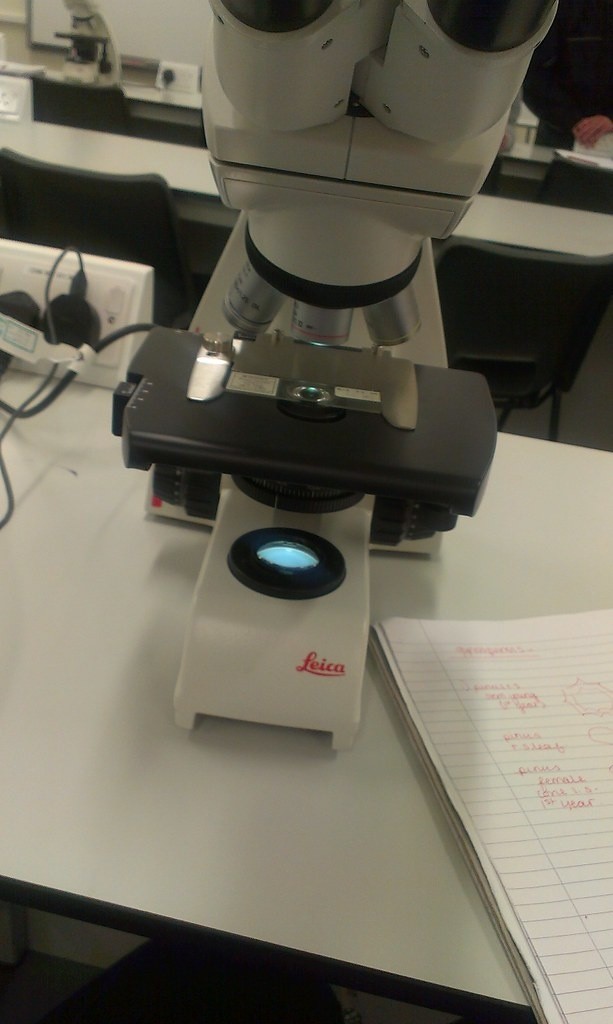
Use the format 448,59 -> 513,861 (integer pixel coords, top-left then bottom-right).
26,0 -> 211,76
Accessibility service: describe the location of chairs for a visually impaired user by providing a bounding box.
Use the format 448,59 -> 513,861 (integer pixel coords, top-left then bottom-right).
430,236 -> 613,440
0,148 -> 191,325
540,155 -> 613,216
28,73 -> 125,137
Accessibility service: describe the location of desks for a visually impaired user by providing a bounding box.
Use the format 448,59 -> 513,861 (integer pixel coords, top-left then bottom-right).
0,0 -> 613,1024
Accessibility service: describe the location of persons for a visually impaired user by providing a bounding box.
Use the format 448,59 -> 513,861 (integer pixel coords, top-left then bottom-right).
522,0 -> 612,157
